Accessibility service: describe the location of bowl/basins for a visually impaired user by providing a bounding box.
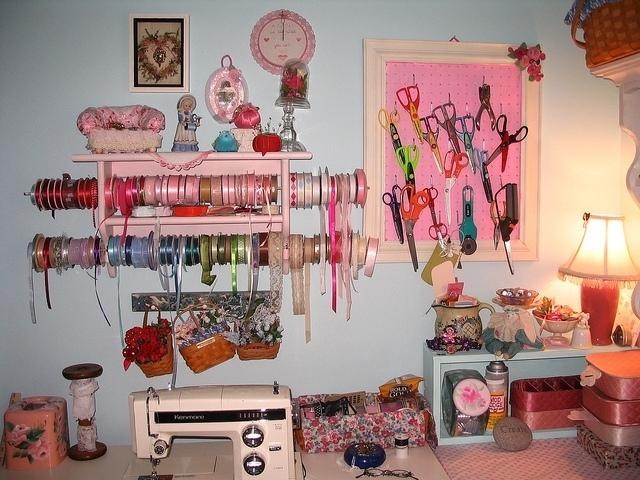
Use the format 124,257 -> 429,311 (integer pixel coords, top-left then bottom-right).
496,287 -> 539,306
344,443 -> 386,468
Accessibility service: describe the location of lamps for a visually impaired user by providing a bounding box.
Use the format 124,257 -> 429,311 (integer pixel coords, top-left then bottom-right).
558,213 -> 638,344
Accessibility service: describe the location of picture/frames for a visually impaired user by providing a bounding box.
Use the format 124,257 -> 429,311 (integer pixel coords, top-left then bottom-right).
128,12 -> 191,94
362,38 -> 540,262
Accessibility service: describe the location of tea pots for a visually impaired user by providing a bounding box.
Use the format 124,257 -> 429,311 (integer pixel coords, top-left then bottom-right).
431,298 -> 496,345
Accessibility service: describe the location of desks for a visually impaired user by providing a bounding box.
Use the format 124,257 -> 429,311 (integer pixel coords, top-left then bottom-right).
423,343 -> 640,448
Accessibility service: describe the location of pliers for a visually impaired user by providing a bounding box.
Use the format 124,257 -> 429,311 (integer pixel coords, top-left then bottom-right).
490,200 -> 507,250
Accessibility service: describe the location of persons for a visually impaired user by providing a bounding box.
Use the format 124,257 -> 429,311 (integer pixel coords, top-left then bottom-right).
171,94 -> 200,151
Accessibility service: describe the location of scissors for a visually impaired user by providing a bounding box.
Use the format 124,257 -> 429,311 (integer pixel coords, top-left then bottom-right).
379,86 -> 530,271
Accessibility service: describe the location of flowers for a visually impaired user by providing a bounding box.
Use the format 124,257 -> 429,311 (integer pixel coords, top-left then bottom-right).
506,42 -> 545,81
122,298 -> 284,372
280,62 -> 308,100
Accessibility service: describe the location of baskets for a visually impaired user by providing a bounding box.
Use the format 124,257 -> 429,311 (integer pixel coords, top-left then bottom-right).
172,303 -> 236,374
237,341 -> 280,360
571,0 -> 640,69
134,304 -> 174,378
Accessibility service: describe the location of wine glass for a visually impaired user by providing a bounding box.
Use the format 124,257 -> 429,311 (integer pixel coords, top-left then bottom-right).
533,311 -> 583,348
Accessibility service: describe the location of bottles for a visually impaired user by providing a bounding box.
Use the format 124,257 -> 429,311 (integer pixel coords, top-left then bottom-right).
479,360 -> 510,433
